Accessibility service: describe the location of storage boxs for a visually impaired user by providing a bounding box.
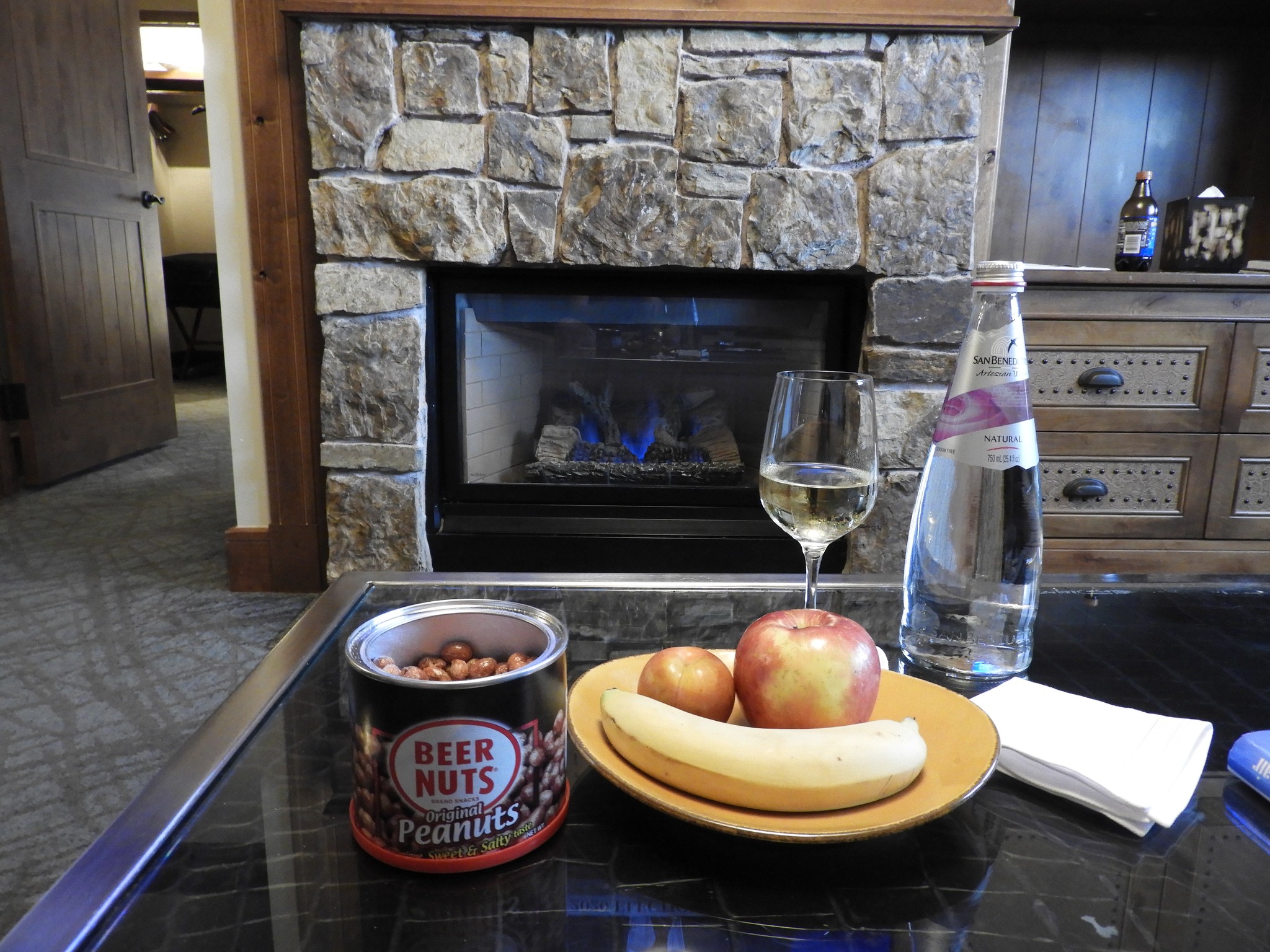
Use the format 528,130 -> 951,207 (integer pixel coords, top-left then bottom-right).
1159,198 -> 1250,272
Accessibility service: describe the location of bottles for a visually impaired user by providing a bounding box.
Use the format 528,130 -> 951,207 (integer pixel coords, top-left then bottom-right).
1115,171 -> 1158,271
899,261 -> 1044,682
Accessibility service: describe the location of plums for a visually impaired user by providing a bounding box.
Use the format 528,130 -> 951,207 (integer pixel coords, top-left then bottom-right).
637,647 -> 735,724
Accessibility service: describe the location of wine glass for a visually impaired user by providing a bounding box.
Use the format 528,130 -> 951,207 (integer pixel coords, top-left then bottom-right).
759,369 -> 879,608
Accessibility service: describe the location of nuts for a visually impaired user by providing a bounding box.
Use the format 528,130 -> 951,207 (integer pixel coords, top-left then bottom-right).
372,640 -> 538,681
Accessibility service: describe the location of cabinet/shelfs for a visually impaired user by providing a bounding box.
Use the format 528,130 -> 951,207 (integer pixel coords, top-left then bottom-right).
1021,319 -> 1270,576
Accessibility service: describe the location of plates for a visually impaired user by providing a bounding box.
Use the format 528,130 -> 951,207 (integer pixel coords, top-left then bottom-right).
569,649 -> 1000,843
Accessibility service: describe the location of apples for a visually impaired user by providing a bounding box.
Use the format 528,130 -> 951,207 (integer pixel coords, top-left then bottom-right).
732,609 -> 881,730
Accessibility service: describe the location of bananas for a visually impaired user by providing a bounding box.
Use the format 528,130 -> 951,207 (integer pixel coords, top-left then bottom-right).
600,688 -> 925,813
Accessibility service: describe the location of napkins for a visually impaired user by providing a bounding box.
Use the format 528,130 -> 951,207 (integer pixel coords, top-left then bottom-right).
968,677 -> 1214,838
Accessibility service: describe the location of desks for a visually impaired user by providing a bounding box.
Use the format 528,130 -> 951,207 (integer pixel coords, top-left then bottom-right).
0,570 -> 1270,952
